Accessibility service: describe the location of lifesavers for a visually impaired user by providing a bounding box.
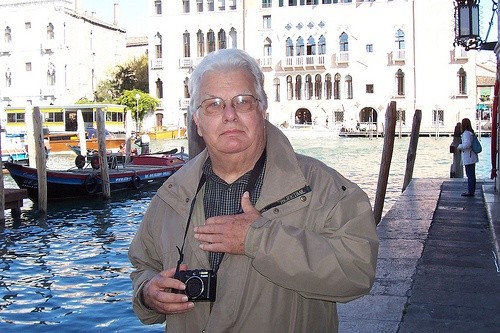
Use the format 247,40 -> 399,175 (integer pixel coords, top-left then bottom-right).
84,176 -> 98,194
132,176 -> 141,189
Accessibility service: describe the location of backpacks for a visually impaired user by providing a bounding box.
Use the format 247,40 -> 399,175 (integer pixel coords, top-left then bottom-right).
470,133 -> 482,161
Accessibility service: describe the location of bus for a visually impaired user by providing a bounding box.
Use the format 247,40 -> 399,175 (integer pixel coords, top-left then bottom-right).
3,103 -> 128,137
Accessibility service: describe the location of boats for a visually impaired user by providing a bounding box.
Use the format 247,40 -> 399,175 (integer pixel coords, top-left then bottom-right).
0,132 -> 190,203
136,93 -> 141,140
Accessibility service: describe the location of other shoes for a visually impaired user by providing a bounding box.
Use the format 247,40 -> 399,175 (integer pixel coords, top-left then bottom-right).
461,191 -> 474,196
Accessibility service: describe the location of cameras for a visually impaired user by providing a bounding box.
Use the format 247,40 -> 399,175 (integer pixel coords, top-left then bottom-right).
172,269 -> 215,302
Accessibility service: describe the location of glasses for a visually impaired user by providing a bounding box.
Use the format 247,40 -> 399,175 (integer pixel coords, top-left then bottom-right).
193,93 -> 263,113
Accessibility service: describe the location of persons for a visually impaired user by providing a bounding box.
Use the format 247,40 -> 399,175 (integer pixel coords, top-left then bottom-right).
131,131 -> 150,156
457,118 -> 482,197
280,121 -> 287,129
342,118 -> 360,133
481,108 -> 493,120
295,114 -> 307,125
326,118 -> 328,128
44,137 -> 51,168
275,111 -> 378,137
